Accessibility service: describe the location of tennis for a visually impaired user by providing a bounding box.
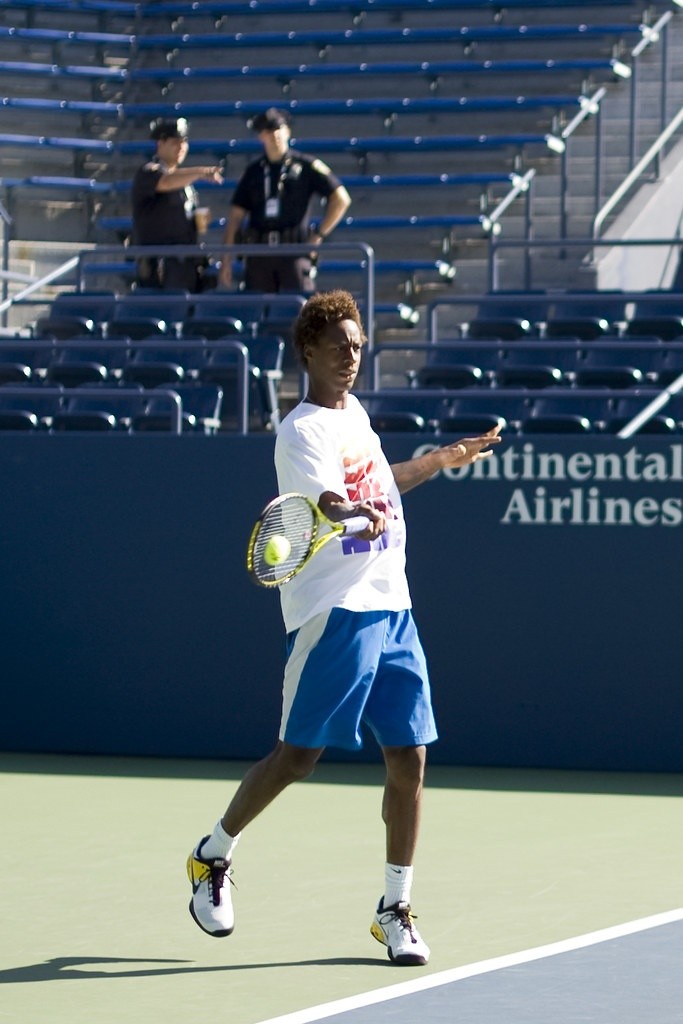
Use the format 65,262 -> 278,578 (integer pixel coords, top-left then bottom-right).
263,536 -> 291,566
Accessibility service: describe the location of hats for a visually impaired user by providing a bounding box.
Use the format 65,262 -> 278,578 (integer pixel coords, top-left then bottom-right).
251,107 -> 285,130
150,118 -> 187,140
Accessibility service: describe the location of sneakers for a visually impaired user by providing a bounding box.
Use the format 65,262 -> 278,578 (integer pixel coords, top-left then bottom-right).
370,896 -> 430,965
187,836 -> 238,937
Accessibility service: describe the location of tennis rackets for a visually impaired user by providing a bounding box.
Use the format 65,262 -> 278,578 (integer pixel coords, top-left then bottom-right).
247,494 -> 371,588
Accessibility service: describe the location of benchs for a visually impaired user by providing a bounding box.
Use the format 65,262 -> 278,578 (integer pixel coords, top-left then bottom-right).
0,0 -> 681,330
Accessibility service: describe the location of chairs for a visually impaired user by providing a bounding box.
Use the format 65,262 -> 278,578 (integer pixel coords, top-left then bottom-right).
0,315 -> 683,434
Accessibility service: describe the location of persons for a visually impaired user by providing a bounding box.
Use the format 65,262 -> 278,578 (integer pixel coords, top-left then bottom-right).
129,117 -> 227,292
186,294 -> 508,969
221,104 -> 350,295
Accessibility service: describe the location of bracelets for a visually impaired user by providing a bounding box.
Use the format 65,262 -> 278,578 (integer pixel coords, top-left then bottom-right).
316,230 -> 327,239
